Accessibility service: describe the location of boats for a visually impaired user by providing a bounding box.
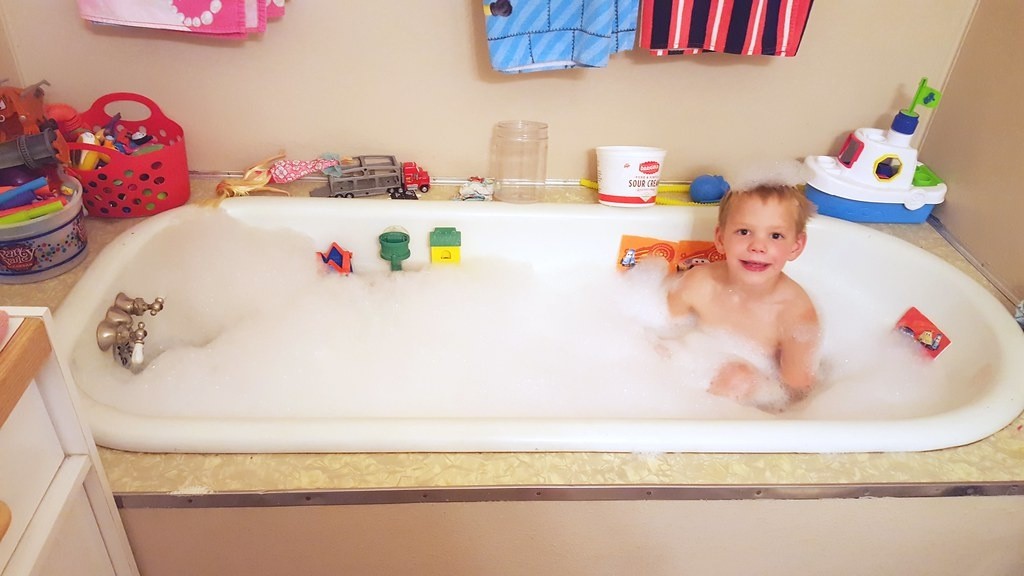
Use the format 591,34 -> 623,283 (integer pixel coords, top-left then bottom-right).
799,77 -> 949,224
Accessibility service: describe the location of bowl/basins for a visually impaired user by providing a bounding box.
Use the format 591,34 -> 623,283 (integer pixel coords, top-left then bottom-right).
0,174 -> 88,285
597,145 -> 665,207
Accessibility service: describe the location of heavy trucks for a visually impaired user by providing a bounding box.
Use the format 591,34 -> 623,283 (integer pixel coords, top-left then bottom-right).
309,154 -> 430,198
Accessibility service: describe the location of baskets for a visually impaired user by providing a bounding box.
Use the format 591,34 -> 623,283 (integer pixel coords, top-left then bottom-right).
63,93 -> 192,220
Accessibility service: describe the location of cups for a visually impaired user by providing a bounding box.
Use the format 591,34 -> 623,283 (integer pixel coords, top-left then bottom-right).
495,120 -> 549,204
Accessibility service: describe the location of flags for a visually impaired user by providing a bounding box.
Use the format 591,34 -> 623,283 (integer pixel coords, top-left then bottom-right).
916,86 -> 941,108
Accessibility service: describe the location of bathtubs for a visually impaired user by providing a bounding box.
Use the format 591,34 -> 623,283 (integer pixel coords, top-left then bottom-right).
48,189 -> 1024,452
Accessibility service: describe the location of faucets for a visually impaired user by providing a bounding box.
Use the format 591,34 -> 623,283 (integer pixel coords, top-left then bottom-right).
106,306 -> 134,328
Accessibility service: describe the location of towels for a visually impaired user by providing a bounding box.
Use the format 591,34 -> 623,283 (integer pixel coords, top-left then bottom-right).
481,1 -> 814,76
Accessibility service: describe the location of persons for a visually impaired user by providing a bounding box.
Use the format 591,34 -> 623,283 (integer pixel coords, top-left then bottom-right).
667,184 -> 820,389
